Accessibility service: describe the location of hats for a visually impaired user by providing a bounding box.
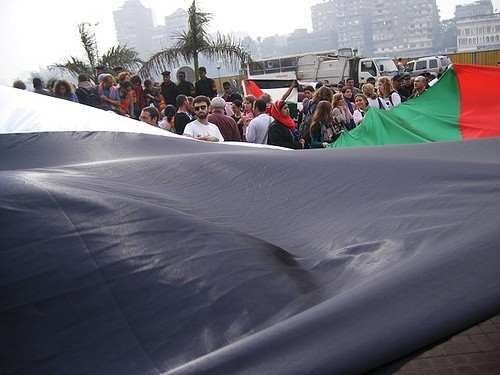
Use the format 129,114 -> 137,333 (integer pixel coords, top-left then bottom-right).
33,78 -> 43,84
161,71 -> 171,75
404,74 -> 411,80
211,97 -> 226,107
393,75 -> 404,81
223,81 -> 231,89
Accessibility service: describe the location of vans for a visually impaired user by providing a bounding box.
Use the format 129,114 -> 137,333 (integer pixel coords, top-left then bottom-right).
404,56 -> 453,89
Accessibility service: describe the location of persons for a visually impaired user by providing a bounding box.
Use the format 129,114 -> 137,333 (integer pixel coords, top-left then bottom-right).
14,56 -> 446,150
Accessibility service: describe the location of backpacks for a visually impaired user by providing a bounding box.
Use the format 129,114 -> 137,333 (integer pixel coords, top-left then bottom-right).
389,90 -> 408,108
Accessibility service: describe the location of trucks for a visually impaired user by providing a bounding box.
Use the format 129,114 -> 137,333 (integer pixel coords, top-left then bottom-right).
295,54 -> 399,97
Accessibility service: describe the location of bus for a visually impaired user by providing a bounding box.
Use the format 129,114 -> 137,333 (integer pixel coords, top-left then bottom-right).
239,47 -> 353,77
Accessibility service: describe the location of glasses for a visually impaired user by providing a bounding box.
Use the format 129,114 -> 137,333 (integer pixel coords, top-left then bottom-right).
193,105 -> 206,111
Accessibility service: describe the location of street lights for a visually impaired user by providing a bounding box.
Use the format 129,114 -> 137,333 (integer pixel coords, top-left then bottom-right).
86,22 -> 100,59
